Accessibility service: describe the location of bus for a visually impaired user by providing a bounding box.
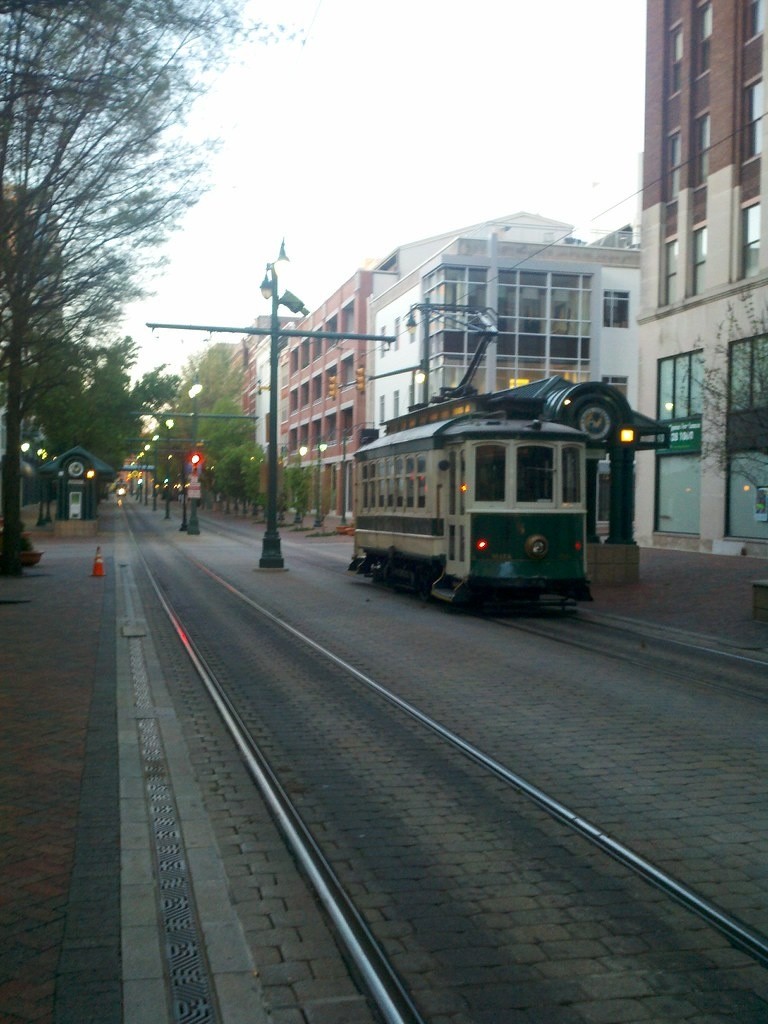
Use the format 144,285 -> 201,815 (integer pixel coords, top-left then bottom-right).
347,394 -> 597,619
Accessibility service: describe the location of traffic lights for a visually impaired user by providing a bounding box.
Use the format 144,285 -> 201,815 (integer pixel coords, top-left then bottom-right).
191,453 -> 200,466
329,376 -> 335,399
356,369 -> 365,391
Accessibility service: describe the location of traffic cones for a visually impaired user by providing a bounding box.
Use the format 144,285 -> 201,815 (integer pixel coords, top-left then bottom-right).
91,548 -> 106,577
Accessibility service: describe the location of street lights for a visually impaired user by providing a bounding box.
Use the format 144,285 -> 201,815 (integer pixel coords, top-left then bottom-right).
293,443 -> 308,524
314,437 -> 328,528
130,421 -> 172,521
20,433 -> 54,526
261,263 -> 286,569
187,374 -> 203,537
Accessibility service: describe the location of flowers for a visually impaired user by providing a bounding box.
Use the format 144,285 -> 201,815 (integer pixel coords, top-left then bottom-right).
19,531 -> 33,551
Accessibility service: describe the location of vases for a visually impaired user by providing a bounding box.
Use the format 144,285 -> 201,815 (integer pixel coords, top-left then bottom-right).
336,526 -> 355,536
20,551 -> 44,566
